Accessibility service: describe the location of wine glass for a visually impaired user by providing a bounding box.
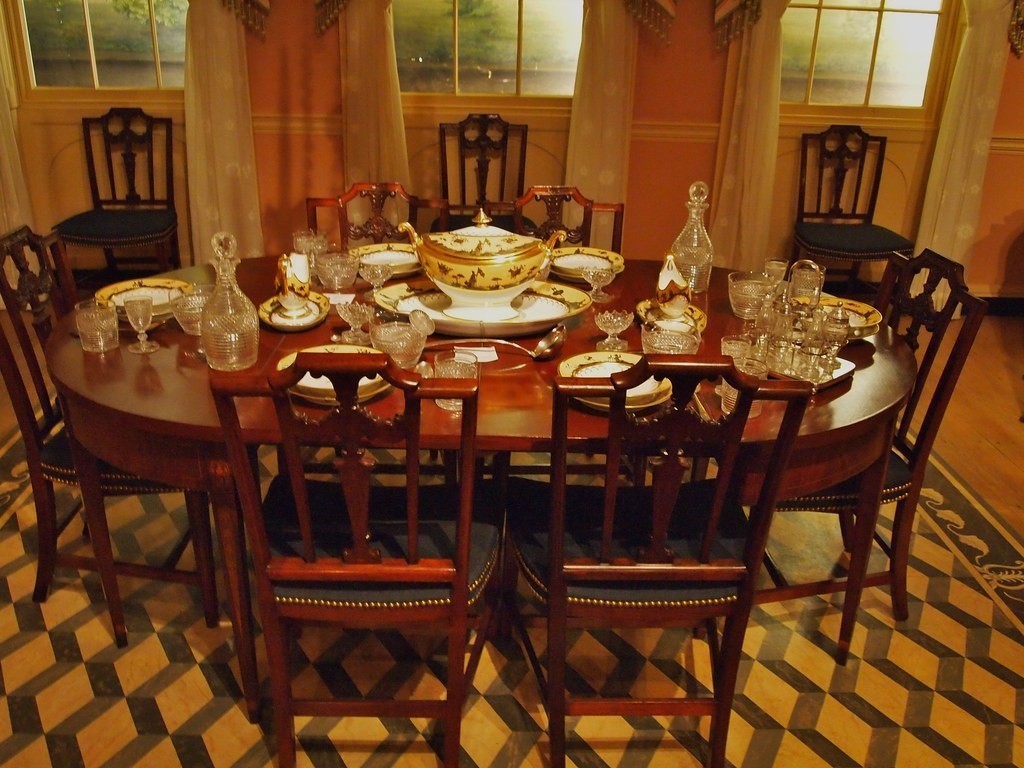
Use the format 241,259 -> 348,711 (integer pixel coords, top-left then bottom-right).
123,295 -> 159,354
576,266 -> 615,302
331,301 -> 374,345
594,310 -> 634,351
359,260 -> 394,301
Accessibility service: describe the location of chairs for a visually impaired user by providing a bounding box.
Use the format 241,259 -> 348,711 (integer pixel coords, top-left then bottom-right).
504,355 -> 811,768
0,219 -> 264,627
787,126 -> 916,295
307,182 -> 449,252
209,350 -> 507,768
511,185 -> 626,256
432,112 -> 530,234
54,107 -> 181,285
689,250 -> 989,665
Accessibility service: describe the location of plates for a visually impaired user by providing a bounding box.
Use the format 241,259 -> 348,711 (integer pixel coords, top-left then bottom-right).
374,281 -> 592,337
258,291 -> 330,330
95,278 -> 193,323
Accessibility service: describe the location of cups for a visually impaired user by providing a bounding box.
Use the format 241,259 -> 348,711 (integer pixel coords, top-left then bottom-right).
275,252 -> 312,319
369,311 -> 427,369
641,319 -> 702,354
434,350 -> 477,411
315,246 -> 360,290
292,230 -> 327,276
75,298 -> 119,352
714,257 -> 849,417
168,283 -> 215,335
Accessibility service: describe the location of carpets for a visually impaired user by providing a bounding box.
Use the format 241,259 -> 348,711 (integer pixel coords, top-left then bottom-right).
0,415 -> 1024,768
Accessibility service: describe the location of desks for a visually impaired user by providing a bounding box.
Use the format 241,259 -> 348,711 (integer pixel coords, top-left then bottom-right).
44,245 -> 916,623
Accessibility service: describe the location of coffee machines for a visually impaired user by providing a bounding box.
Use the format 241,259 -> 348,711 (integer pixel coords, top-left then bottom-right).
671,181 -> 714,293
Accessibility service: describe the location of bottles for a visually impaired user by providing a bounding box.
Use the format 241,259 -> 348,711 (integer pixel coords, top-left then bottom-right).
656,256 -> 690,317
201,231 -> 260,371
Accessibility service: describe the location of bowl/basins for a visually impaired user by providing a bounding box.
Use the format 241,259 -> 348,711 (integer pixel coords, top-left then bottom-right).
847,324 -> 879,338
789,295 -> 882,328
398,208 -> 567,321
349,243 -> 422,278
550,247 -> 625,282
275,344 -> 391,406
557,299 -> 707,412
